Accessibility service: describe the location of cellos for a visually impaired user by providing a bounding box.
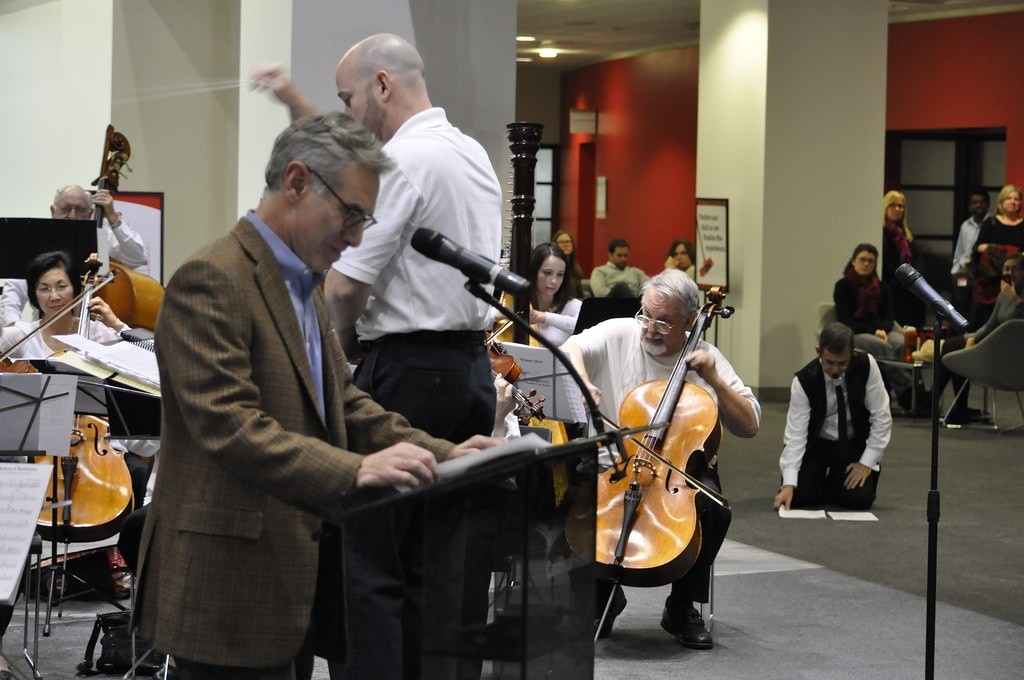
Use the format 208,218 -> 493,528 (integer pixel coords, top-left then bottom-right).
34,250 -> 135,619
566,286 -> 735,642
85,122 -> 166,332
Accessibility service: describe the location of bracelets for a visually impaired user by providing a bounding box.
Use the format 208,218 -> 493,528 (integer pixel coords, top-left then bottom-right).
109,217 -> 121,228
112,319 -> 120,330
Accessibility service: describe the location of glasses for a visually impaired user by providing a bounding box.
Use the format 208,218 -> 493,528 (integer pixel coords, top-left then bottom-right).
306,164 -> 375,230
634,306 -> 679,335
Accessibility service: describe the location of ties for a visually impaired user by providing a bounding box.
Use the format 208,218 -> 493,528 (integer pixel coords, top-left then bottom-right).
834,377 -> 849,461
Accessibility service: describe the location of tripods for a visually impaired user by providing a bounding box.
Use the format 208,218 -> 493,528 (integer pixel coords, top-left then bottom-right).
43,454 -> 131,637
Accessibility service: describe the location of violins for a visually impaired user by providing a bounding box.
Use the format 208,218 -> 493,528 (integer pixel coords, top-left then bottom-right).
485,331 -> 547,426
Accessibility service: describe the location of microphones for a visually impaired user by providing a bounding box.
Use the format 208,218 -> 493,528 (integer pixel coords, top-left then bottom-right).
896,262 -> 970,334
410,228 -> 530,296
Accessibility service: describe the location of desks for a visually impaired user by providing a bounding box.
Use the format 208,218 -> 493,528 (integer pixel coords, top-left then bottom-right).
883,358 -> 992,426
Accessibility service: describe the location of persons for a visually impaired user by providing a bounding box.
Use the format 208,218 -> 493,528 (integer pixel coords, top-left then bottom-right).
252,33 -> 502,680
0,185 -> 160,680
493,186 -> 1024,649
157,112 -> 510,680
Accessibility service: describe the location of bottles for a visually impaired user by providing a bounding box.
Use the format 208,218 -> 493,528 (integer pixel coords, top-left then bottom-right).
903,325 -> 917,362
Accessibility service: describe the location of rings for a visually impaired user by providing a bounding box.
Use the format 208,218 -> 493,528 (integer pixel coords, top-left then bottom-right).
25,369 -> 29,373
97,315 -> 103,321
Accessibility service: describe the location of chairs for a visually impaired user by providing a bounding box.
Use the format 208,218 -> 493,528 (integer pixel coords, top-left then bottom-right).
939,319 -> 1024,434
814,301 -> 836,344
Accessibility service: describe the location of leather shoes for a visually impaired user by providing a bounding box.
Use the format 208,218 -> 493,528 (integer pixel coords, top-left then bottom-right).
660,596 -> 714,648
594,583 -> 626,640
